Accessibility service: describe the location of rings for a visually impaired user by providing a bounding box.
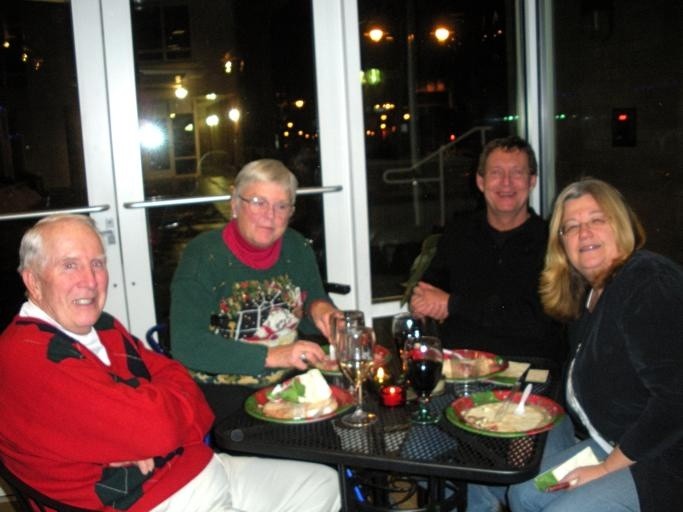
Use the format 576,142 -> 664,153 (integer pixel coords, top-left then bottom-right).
300,352 -> 305,357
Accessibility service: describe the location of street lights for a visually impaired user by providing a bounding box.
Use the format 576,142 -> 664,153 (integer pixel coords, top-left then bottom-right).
180,106 -> 244,167
363,21 -> 460,222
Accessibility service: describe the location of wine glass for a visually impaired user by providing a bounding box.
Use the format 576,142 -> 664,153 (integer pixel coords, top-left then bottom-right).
328,308 -> 379,430
390,312 -> 444,426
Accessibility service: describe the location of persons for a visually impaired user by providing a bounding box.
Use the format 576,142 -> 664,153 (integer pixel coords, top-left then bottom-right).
505,175 -> 682,511
0,213 -> 344,511
400,133 -> 570,511
166,159 -> 360,511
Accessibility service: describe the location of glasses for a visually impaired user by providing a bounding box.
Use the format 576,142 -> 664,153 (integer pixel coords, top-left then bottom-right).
235,194 -> 293,219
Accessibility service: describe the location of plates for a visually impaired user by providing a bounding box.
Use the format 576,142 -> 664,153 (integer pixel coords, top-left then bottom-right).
440,347 -> 510,383
303,340 -> 393,377
242,380 -> 356,427
443,389 -> 566,439
